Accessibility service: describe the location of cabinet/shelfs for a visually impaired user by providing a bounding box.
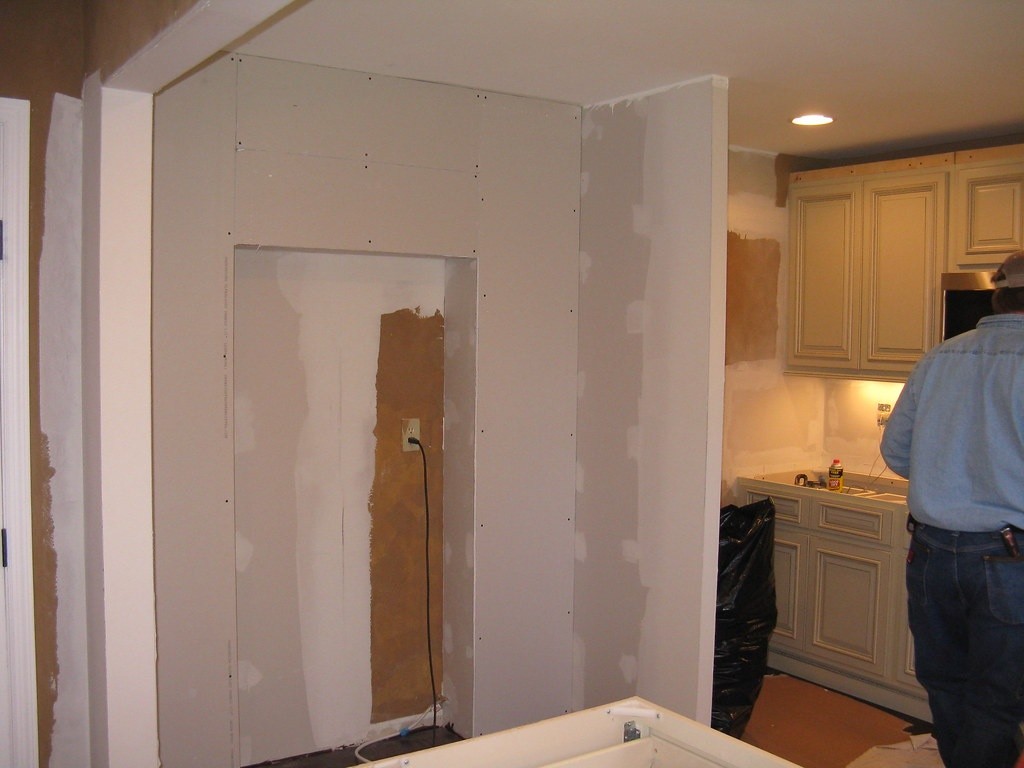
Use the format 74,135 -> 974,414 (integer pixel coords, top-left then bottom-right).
779,151 -> 1023,384
743,487 -> 933,725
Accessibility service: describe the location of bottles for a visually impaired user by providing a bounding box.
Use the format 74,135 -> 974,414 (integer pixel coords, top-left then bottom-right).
827,459 -> 843,493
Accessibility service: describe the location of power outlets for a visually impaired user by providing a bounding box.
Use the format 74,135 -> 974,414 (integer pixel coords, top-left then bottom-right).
402,418 -> 421,452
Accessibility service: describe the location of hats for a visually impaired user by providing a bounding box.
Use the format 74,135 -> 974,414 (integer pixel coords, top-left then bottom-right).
994,250 -> 1024,290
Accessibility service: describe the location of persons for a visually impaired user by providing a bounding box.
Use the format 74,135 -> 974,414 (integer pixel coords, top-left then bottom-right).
880,252 -> 1024,768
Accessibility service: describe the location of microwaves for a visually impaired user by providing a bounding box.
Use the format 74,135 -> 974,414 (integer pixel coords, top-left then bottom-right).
940,271 -> 997,344
